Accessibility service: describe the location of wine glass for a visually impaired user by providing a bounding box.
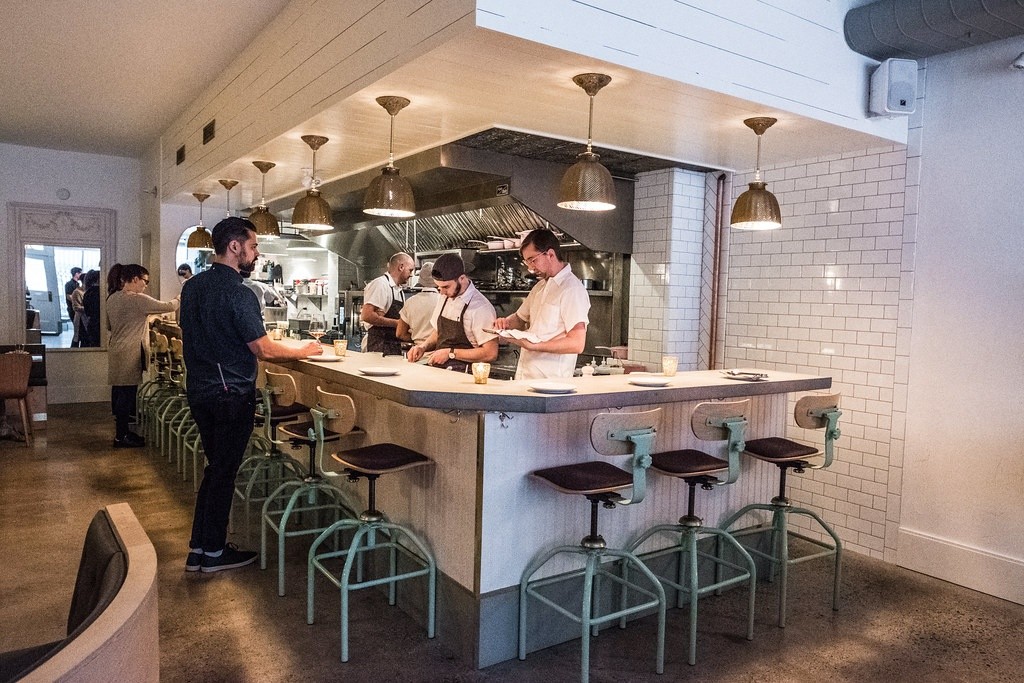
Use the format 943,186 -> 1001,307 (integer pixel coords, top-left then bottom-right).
308,314 -> 326,342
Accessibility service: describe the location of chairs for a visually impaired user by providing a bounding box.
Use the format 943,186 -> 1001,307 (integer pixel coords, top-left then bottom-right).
0,348 -> 36,449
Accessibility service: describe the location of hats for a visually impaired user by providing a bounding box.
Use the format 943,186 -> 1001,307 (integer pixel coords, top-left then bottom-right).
432,254 -> 475,280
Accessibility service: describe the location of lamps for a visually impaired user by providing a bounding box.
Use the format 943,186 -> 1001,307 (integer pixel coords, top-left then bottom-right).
729,116 -> 783,234
552,72 -> 620,214
291,134 -> 336,231
186,192 -> 213,251
360,95 -> 418,219
247,160 -> 281,240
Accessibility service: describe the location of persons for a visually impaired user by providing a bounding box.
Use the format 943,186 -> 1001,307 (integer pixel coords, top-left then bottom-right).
406,252 -> 498,375
360,252 -> 414,358
240,270 -> 280,331
65,266 -> 100,348
179,217 -> 323,573
178,263 -> 195,283
105,262 -> 182,448
397,262 -> 443,366
492,229 -> 591,382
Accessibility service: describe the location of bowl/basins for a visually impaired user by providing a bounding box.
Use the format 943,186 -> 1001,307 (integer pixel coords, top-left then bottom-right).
581,279 -> 594,289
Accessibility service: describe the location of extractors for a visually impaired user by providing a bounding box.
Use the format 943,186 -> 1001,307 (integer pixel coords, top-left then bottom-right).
273,144 -> 635,269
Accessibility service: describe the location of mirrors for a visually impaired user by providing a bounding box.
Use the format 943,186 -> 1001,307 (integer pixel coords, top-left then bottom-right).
23,243 -> 102,350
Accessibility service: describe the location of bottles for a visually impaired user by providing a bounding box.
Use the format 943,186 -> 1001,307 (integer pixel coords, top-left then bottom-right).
597,355 -> 610,375
296,279 -> 328,295
581,363 -> 594,377
590,356 -> 599,375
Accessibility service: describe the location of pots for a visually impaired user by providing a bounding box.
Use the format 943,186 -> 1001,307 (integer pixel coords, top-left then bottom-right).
595,345 -> 627,359
468,235 -> 521,250
515,229 -> 563,244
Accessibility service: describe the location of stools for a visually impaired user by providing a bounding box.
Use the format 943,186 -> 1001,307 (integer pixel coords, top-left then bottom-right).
134,329 -> 437,665
618,397 -> 759,668
714,387 -> 849,632
513,404 -> 671,683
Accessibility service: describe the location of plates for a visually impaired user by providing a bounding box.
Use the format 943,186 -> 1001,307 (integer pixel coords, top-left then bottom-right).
365,352 -> 403,360
526,382 -> 578,394
630,377 -> 671,387
720,370 -> 770,380
307,355 -> 343,362
358,367 -> 400,376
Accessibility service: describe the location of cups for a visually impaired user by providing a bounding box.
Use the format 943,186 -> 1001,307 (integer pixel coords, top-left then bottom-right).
608,367 -> 625,374
15,344 -> 24,352
471,363 -> 491,384
351,285 -> 357,291
663,356 -> 679,376
334,340 -> 348,357
273,329 -> 283,340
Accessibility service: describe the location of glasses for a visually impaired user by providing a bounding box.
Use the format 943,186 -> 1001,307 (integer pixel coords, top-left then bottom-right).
134,275 -> 149,285
521,250 -> 548,266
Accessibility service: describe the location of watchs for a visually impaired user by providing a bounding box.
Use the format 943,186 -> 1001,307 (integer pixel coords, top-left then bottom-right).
448,347 -> 456,359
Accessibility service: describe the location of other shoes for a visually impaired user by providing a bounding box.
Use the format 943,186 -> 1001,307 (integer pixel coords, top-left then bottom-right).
200,548 -> 259,571
113,431 -> 145,448
184,551 -> 202,571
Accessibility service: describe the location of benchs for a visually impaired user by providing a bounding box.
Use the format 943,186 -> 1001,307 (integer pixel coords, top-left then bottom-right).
0,500 -> 163,683
